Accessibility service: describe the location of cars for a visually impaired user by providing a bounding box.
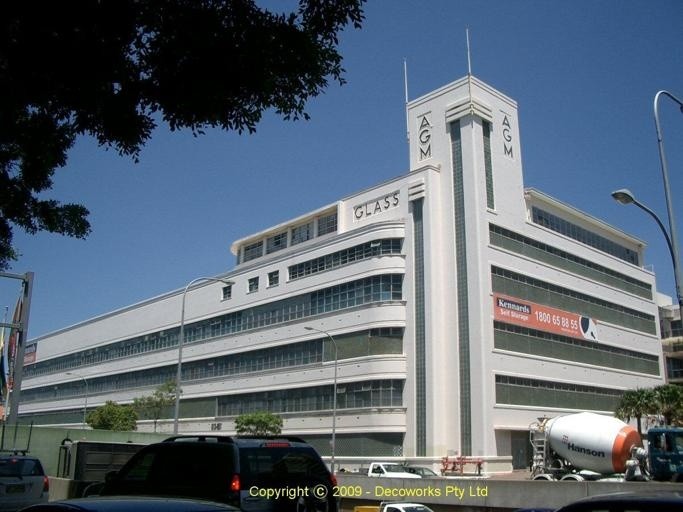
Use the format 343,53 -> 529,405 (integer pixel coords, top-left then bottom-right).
2,495 -> 242,511
554,489 -> 683,511
405,465 -> 437,477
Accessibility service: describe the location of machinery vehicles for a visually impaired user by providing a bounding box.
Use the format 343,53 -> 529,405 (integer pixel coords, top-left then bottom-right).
530,413 -> 682,483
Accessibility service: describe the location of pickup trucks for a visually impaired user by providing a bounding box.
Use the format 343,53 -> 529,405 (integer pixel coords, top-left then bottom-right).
336,462 -> 422,477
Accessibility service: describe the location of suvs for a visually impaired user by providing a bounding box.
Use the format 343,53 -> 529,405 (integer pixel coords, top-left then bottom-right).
46,438 -> 148,502
98,433 -> 339,510
0,448 -> 49,512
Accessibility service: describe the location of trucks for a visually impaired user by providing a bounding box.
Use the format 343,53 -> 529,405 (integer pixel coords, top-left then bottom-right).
354,501 -> 432,512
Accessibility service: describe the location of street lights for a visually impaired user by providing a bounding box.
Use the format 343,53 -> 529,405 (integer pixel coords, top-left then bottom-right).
66,372 -> 88,428
610,89 -> 682,315
173,276 -> 236,437
304,325 -> 337,478
